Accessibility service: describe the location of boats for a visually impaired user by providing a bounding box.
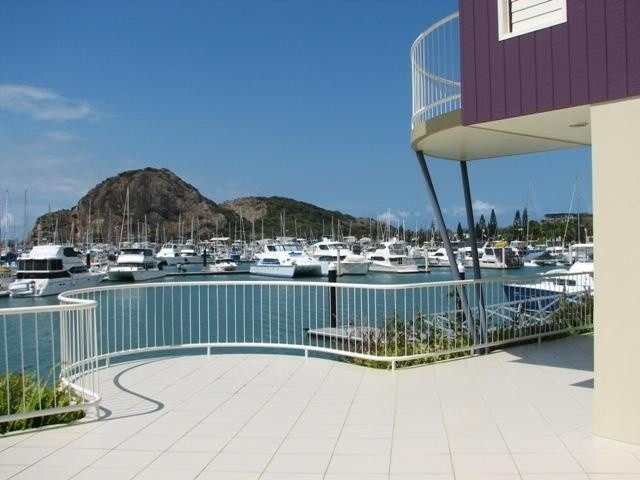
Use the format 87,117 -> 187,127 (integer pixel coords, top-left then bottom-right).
501,242 -> 593,314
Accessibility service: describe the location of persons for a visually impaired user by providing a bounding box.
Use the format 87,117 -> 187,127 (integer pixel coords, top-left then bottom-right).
177,261 -> 187,272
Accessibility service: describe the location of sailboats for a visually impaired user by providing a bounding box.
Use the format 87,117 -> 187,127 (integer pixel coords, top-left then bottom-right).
200,205 -> 376,278
369,207 -> 572,273
0,186 -> 203,297
